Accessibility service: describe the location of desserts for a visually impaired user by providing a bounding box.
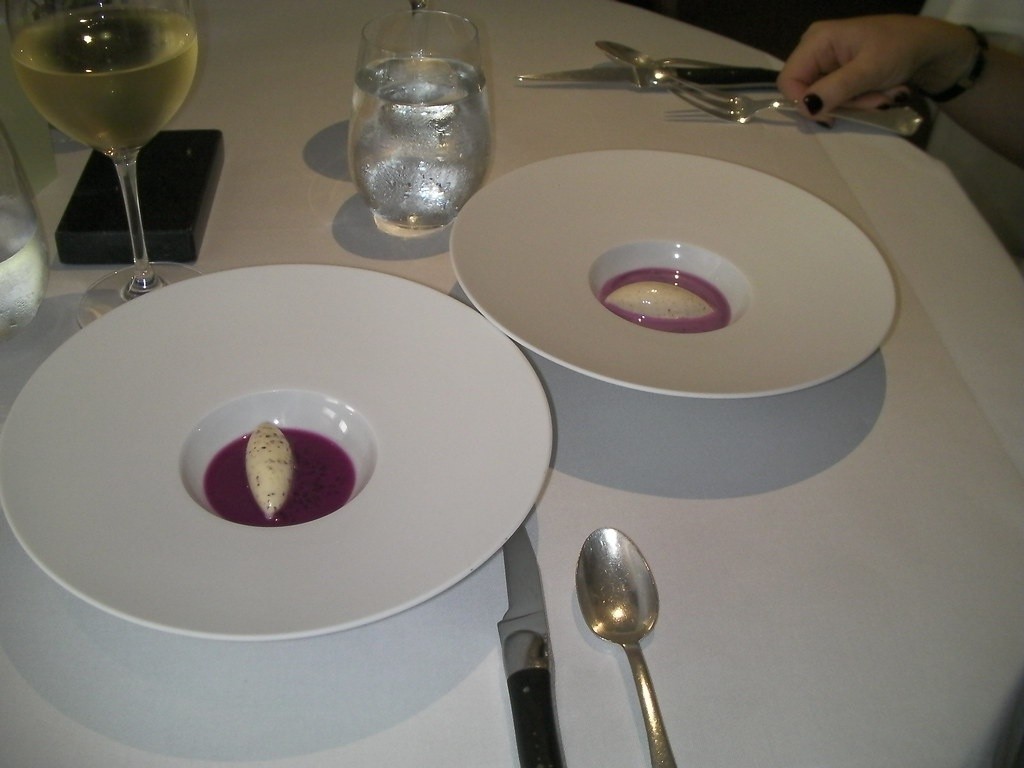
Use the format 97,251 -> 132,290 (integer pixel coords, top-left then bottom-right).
203,420 -> 354,527
598,267 -> 731,334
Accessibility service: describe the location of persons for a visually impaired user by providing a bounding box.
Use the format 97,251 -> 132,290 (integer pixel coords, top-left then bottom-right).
777,15 -> 1024,168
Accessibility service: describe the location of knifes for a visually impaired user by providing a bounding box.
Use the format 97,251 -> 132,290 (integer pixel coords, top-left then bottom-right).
497,525 -> 564,768
518,66 -> 783,85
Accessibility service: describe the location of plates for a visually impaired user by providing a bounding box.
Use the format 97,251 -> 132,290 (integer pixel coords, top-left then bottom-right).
450,150 -> 895,398
0,264 -> 554,639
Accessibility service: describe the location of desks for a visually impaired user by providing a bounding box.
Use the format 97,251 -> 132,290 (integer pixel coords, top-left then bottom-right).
0,0 -> 1024,768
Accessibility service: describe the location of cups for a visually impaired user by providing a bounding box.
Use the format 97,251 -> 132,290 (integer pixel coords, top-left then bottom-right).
0,126 -> 49,343
350,10 -> 492,235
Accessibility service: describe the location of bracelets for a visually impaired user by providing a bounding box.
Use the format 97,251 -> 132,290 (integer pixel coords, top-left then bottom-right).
910,24 -> 990,102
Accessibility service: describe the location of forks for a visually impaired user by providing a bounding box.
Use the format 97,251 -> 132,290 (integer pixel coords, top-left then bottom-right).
655,75 -> 922,136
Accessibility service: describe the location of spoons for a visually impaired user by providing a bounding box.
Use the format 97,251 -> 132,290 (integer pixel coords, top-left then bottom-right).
577,528 -> 677,768
595,38 -> 736,67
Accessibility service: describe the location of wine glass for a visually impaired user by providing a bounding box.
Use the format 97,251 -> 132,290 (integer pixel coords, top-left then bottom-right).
4,0 -> 204,331
